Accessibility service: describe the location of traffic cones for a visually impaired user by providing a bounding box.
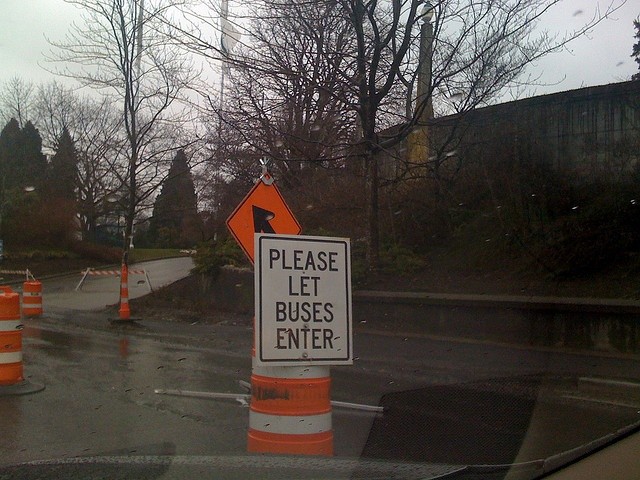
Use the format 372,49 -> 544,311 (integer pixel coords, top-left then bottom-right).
75,266 -> 153,292
111,264 -> 143,323
0,269 -> 36,282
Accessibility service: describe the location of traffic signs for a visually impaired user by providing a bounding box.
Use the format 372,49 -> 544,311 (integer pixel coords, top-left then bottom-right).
254,232 -> 355,365
226,170 -> 304,268
